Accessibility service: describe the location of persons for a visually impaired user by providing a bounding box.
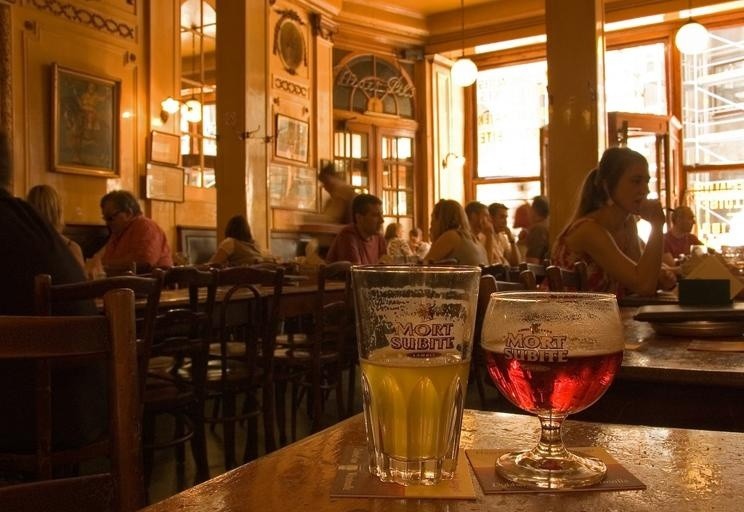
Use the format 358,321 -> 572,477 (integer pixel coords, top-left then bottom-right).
91,190 -> 174,275
526,196 -> 547,266
0,132 -> 111,481
26,183 -> 87,314
326,195 -> 387,269
383,221 -> 414,265
302,161 -> 357,226
70,83 -> 109,165
464,203 -> 506,267
488,202 -> 520,264
663,206 -> 708,276
550,147 -> 675,301
407,228 -> 431,263
196,214 -> 269,272
426,200 -> 490,271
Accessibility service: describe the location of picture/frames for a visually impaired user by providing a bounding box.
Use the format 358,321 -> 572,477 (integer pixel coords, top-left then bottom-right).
146,162 -> 184,203
275,112 -> 309,163
150,130 -> 180,165
49,63 -> 123,178
269,160 -> 317,211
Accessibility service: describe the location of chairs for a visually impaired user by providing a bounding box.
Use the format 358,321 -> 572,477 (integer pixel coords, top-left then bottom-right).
141,264 -> 212,378
205,264 -> 278,433
481,270 -> 537,319
519,259 -> 553,276
1,288 -> 144,512
137,267 -> 220,507
274,263 -> 314,410
546,259 -> 588,293
103,260 -> 137,277
469,263 -> 508,410
176,266 -> 285,476
0,274 -> 163,481
272,261 -> 356,447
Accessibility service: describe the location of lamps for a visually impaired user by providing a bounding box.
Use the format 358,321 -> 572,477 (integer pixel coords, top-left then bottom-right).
182,23 -> 203,122
442,152 -> 462,169
449,1 -> 478,87
675,0 -> 710,55
160,97 -> 180,123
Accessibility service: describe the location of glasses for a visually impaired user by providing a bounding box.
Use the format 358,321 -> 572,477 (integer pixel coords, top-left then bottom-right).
103,211 -> 121,221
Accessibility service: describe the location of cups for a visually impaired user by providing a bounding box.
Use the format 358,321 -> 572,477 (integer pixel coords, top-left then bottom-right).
350,264 -> 479,486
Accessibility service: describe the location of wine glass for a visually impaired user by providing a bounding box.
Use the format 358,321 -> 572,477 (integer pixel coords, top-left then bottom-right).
480,289 -> 624,493
721,245 -> 743,276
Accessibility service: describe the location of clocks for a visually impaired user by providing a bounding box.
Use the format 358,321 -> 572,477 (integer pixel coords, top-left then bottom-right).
278,19 -> 307,75
